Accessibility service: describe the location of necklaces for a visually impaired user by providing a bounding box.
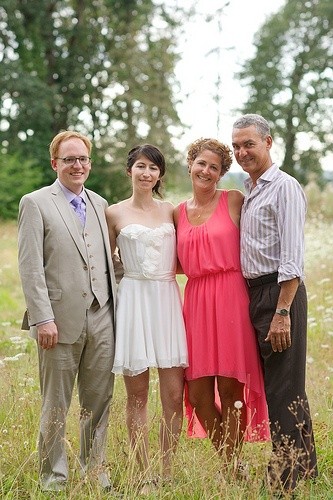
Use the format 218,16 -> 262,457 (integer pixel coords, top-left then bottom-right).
198,213 -> 202,219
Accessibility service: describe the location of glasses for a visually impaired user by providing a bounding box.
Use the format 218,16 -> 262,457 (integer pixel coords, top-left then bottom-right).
56,155 -> 92,165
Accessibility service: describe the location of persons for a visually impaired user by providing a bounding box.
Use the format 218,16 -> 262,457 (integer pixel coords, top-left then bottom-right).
105,144 -> 190,496
17,132 -> 118,493
172,138 -> 271,486
231,113 -> 319,500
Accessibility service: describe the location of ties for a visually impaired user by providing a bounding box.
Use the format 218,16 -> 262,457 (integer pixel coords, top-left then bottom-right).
72,196 -> 85,225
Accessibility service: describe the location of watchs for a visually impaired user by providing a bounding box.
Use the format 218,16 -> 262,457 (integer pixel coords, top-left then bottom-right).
276,308 -> 291,316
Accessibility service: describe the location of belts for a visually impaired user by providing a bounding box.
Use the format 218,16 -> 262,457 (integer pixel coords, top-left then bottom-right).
246,272 -> 279,287
